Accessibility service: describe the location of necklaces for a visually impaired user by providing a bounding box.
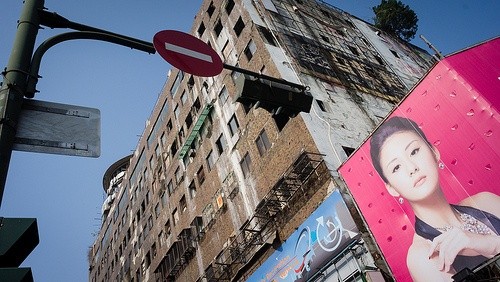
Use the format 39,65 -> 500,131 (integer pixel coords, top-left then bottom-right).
432,206 -> 491,236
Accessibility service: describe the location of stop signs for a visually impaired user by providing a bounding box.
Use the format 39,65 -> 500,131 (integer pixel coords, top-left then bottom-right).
153,30 -> 224,77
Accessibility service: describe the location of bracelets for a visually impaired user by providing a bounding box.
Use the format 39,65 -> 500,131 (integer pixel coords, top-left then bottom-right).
488,236 -> 500,258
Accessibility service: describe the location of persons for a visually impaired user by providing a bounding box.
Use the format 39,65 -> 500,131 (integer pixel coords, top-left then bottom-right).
369,116 -> 500,282
